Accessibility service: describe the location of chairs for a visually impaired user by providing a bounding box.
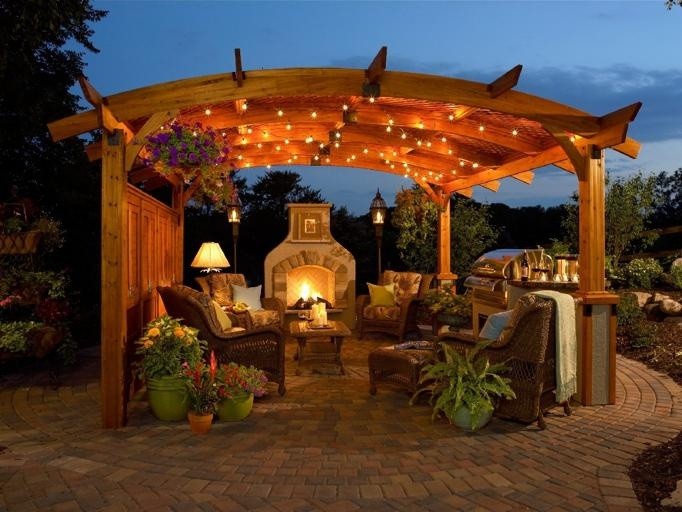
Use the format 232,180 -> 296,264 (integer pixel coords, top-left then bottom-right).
196,273 -> 284,328
154,284 -> 286,397
355,269 -> 433,344
434,290 -> 576,430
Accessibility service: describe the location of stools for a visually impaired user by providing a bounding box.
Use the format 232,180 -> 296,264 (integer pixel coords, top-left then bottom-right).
367,339 -> 437,404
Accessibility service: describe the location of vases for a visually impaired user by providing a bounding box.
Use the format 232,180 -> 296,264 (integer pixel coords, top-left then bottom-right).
144,373 -> 254,434
436,313 -> 471,332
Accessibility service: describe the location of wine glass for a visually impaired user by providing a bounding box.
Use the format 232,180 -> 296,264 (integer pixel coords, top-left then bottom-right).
531,261 -> 543,281
542,261 -> 551,280
296,309 -> 314,331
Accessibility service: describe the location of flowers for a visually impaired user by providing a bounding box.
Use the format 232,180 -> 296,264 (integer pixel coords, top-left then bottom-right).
135,313 -> 266,415
424,282 -> 473,318
141,120 -> 239,215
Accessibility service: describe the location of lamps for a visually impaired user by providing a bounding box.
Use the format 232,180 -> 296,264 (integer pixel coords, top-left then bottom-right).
190,241 -> 232,296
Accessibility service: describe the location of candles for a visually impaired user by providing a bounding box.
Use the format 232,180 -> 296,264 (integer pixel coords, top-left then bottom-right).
311,302 -> 328,326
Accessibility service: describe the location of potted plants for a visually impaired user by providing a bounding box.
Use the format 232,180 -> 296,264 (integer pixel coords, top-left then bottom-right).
406,341 -> 518,431
0,215 -> 82,375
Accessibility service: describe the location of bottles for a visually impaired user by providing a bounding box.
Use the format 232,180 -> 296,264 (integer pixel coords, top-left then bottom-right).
520,249 -> 529,282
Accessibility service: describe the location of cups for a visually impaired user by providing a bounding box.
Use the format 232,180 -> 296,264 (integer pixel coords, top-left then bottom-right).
555,254 -> 580,282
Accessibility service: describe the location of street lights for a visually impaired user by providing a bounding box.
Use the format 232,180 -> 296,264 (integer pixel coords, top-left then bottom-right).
225,190 -> 244,275
369,187 -> 388,276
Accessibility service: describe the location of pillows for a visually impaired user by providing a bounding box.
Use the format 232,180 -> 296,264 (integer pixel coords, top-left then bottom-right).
366,282 -> 396,308
229,283 -> 264,311
207,300 -> 232,332
478,308 -> 512,339
